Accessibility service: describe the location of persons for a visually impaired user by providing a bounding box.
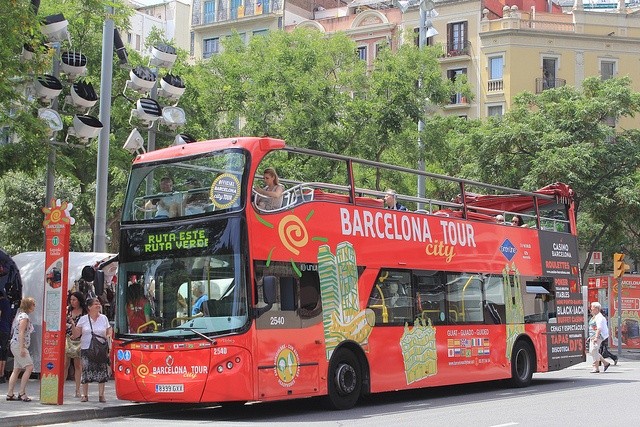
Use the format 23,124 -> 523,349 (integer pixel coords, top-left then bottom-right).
6,297 -> 36,401
145,177 -> 179,220
586,302 -> 610,373
599,309 -> 618,366
495,215 -> 505,224
383,189 -> 407,210
67,297 -> 113,403
0,291 -> 9,379
169,177 -> 210,216
176,292 -> 187,317
183,282 -> 209,318
511,215 -> 529,228
185,297 -> 195,315
125,283 -> 151,333
64,292 -> 87,399
253,167 -> 284,210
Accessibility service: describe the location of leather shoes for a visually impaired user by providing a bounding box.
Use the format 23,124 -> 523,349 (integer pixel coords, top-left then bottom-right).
99,396 -> 106,402
81,396 -> 88,402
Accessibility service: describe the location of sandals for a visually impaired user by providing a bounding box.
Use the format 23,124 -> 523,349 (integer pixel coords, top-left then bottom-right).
18,393 -> 31,402
6,394 -> 22,401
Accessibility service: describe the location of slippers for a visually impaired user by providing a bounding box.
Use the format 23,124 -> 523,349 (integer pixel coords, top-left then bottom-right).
602,363 -> 610,371
590,369 -> 599,373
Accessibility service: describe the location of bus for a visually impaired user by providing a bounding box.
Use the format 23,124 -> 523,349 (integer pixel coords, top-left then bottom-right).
111,136 -> 587,411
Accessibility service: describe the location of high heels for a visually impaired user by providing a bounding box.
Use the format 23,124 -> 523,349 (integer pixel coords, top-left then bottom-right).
75,390 -> 81,398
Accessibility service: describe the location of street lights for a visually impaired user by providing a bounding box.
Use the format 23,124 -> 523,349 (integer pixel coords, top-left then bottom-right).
7,13 -> 104,252
122,40 -> 187,222
396,1 -> 438,214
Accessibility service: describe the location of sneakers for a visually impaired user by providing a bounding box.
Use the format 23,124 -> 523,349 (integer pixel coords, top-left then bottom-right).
614,357 -> 619,365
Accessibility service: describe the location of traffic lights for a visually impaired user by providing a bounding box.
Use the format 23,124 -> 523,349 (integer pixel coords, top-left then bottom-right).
613,253 -> 625,280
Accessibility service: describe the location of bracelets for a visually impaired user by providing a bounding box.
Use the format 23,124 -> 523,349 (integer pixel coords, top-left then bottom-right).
177,297 -> 180,299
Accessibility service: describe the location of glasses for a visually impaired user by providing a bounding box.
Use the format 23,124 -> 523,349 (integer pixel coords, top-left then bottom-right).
498,219 -> 503,222
511,221 -> 517,223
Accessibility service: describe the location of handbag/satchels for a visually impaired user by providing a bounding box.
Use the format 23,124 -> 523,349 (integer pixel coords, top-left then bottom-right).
65,332 -> 82,358
88,334 -> 108,363
